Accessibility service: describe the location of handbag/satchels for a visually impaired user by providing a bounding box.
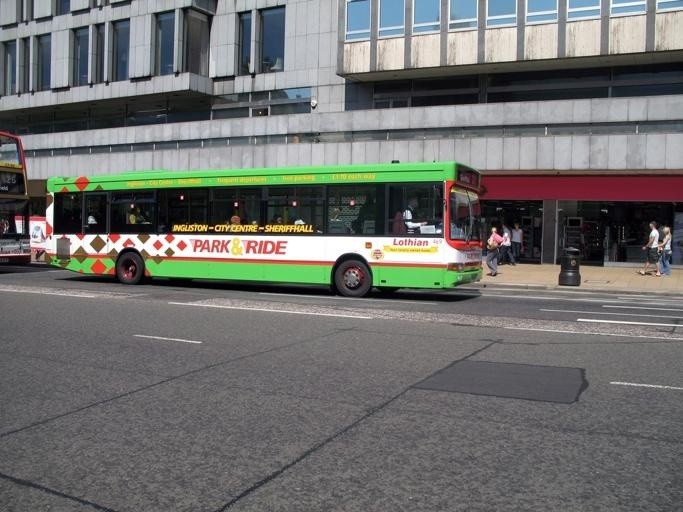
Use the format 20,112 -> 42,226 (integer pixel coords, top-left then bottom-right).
486,241 -> 497,252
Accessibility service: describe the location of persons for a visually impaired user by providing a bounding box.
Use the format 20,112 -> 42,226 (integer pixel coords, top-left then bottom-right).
486,227 -> 498,276
658,225 -> 672,276
130,206 -> 145,224
403,195 -> 427,235
498,224 -> 515,265
638,221 -> 660,277
511,223 -> 523,261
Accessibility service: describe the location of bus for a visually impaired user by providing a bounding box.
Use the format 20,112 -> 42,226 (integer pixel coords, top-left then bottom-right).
41,160 -> 491,303
0,134 -> 34,266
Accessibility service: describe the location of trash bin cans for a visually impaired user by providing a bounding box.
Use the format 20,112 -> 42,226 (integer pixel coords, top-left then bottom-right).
559,246 -> 581,286
